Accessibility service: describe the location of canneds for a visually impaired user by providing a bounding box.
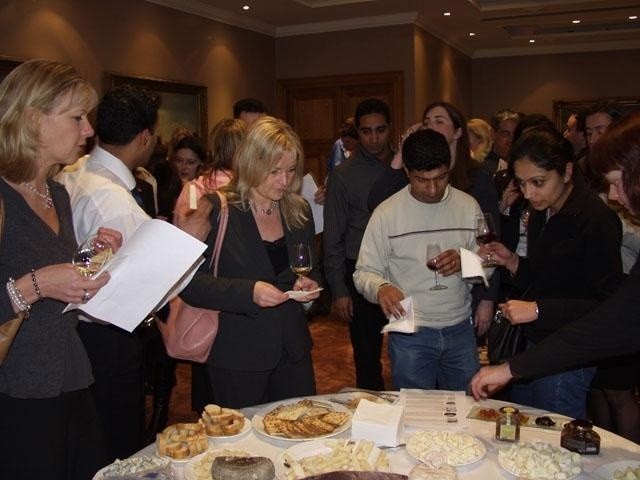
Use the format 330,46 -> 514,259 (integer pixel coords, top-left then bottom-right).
494,407 -> 520,441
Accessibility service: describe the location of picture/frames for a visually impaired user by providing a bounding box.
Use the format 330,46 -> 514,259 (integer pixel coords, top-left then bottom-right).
0,55 -> 25,84
552,98 -> 640,134
104,71 -> 208,160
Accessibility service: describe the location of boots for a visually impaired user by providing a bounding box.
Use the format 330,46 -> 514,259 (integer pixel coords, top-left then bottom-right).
143,386 -> 175,446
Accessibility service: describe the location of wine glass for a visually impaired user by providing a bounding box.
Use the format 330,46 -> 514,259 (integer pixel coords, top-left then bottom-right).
425,242 -> 449,291
474,212 -> 500,267
290,242 -> 315,293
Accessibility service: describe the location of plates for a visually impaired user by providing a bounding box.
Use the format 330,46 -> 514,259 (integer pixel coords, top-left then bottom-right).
94,458 -> 177,480
183,449 -> 262,479
152,438 -> 215,462
195,417 -> 252,441
497,440 -> 583,480
272,441 -> 392,480
328,392 -> 406,413
252,397 -> 354,441
586,459 -> 640,480
406,431 -> 487,468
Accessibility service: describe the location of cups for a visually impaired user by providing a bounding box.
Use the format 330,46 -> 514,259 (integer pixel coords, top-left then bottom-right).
71,234 -> 113,281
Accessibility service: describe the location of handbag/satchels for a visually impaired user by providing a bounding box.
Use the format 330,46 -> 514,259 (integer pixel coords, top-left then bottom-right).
155,294 -> 222,365
0,309 -> 27,368
485,282 -> 535,367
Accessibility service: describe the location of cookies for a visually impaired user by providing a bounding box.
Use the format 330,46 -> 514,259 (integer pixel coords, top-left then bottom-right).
266,398 -> 347,438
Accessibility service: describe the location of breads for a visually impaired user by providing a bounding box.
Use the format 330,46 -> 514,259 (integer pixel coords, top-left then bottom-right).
409,430 -> 481,465
197,404 -> 244,436
612,465 -> 640,480
497,441 -> 582,480
155,423 -> 208,460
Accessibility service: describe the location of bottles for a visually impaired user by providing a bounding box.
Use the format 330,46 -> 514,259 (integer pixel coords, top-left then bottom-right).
560,419 -> 601,457
494,405 -> 522,444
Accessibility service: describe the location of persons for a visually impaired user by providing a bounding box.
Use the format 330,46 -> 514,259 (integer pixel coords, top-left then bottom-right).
233,98 -> 327,206
325,97 -> 394,391
353,127 -> 496,388
46,84 -> 157,463
178,116 -> 326,409
162,120 -> 250,418
368,101 -> 501,337
312,116 -> 358,316
563,103 -> 639,272
134,175 -> 176,446
479,127 -> 623,418
468,110 -> 561,400
144,128 -> 208,218
1,59 -> 123,480
471,126 -> 639,444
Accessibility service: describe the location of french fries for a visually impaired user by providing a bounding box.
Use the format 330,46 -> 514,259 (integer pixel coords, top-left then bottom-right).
368,467 -> 371,468
279,438 -> 389,478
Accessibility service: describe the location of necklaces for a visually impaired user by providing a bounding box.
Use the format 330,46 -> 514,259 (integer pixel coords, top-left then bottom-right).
26,180 -> 55,210
249,198 -> 277,215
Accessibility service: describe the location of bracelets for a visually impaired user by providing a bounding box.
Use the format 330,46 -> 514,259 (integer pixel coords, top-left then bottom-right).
4,278 -> 33,321
30,268 -> 45,303
535,304 -> 538,318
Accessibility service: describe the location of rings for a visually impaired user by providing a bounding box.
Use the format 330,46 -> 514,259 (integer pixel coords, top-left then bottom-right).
82,290 -> 89,300
452,261 -> 456,266
